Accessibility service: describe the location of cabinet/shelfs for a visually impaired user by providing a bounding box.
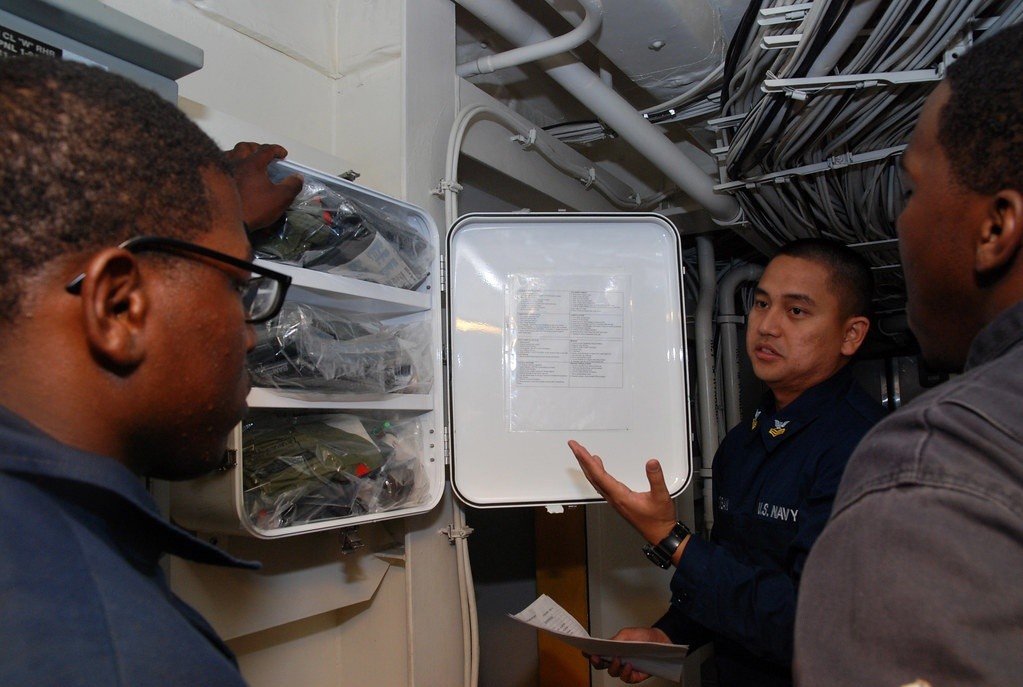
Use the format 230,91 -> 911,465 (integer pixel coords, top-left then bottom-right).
166,157 -> 694,542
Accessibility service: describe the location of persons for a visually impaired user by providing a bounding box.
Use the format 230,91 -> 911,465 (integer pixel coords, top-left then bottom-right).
0,52 -> 303,687
567,236 -> 883,687
791,21 -> 1023,687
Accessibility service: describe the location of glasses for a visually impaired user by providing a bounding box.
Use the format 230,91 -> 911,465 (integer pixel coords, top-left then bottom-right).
65,234 -> 292,324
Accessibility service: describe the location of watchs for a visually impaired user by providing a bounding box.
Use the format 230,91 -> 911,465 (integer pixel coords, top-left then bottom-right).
642,520 -> 690,570
